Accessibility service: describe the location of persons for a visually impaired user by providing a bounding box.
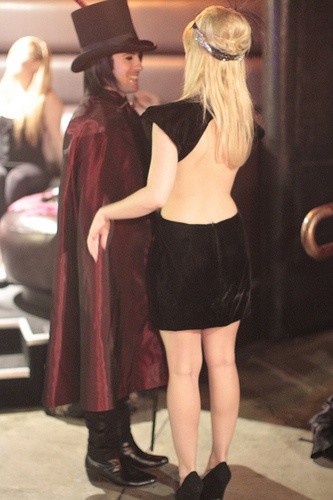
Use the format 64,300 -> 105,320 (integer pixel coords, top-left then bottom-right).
87,6 -> 266,500
46,0 -> 170,488
0,35 -> 65,219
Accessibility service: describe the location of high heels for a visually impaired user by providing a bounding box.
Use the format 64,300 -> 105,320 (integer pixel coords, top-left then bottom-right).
176,471 -> 204,500
202,462 -> 231,500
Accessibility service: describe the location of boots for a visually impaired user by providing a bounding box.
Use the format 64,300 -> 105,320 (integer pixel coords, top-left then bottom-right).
85,410 -> 158,488
116,397 -> 169,470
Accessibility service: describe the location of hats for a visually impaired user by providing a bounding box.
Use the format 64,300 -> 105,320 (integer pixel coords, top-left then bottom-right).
71,0 -> 157,73
